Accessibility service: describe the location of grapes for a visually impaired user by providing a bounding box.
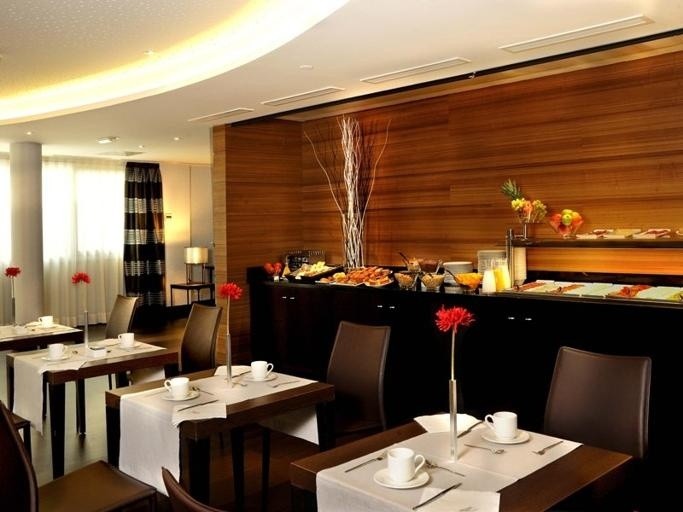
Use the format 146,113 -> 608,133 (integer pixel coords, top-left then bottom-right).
534,200 -> 547,218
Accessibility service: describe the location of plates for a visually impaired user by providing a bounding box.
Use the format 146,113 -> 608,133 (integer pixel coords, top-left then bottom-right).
41,352 -> 73,362
315,270 -> 393,288
38,324 -> 56,329
245,371 -> 277,382
480,429 -> 529,445
165,390 -> 200,402
373,468 -> 431,489
441,261 -> 474,285
119,340 -> 141,349
476,249 -> 506,285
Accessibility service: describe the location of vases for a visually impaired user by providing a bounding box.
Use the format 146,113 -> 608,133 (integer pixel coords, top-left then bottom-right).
226,333 -> 233,388
448,379 -> 458,462
83,310 -> 89,355
11,297 -> 17,328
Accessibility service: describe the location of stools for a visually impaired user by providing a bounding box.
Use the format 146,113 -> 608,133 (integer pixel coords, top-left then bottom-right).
0,403 -> 32,460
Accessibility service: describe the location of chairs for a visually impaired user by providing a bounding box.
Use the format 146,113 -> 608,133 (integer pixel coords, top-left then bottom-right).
542,340 -> 657,510
0,395 -> 160,512
316,319 -> 395,454
179,302 -> 224,376
100,294 -> 141,390
161,467 -> 228,512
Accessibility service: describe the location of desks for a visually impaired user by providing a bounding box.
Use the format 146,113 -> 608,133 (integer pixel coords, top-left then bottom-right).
169,283 -> 216,328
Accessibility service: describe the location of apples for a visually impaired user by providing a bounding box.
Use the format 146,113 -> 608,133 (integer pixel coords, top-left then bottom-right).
263,262 -> 282,273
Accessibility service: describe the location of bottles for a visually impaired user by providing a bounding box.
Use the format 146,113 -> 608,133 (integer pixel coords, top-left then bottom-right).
481,258 -> 513,295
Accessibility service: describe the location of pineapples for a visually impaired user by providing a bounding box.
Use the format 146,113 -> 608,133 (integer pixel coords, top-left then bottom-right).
511,199 -> 524,212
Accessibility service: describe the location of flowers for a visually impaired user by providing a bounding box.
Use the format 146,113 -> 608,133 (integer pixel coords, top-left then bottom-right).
434,302 -> 474,380
4,266 -> 26,325
215,279 -> 243,377
70,272 -> 91,336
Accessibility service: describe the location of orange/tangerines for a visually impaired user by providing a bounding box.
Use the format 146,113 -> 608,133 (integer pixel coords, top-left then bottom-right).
562,209 -> 579,226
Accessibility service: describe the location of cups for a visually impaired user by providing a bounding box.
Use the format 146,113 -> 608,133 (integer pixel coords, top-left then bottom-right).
116,331 -> 134,345
38,314 -> 53,325
163,375 -> 192,398
0,326 -> 16,338
484,410 -> 518,440
47,343 -> 67,355
250,360 -> 274,379
509,246 -> 527,286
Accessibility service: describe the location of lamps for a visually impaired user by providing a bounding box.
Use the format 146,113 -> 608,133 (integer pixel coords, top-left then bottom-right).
183,246 -> 208,285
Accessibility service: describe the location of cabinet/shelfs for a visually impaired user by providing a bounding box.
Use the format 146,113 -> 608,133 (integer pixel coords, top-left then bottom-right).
462,291 -> 683,511
330,285 -> 463,430
245,282 -> 331,456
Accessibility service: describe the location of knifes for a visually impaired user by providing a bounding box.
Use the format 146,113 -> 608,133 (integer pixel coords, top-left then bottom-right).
387,447 -> 424,482
457,420 -> 483,438
411,482 -> 462,510
178,400 -> 218,412
231,370 -> 250,379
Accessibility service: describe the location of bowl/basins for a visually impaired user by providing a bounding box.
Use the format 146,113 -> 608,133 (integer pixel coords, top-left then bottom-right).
452,272 -> 482,291
546,221 -> 581,239
393,257 -> 445,293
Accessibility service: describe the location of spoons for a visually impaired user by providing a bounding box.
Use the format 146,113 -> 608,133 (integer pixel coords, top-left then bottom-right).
426,458 -> 464,478
463,443 -> 504,454
191,385 -> 212,395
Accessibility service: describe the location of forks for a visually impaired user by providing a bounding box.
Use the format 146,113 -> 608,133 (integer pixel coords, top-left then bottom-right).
265,381 -> 299,388
345,448 -> 388,473
532,440 -> 563,456
